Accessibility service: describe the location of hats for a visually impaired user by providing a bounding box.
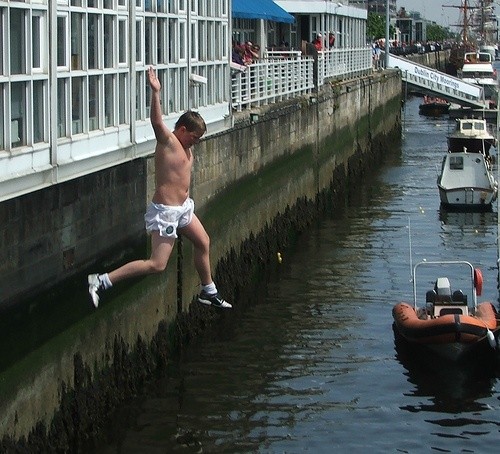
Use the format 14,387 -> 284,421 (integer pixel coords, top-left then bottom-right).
254,44 -> 260,49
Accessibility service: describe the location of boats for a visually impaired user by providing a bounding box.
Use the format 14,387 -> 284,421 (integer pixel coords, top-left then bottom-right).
418,0 -> 500,119
394,261 -> 500,352
437,145 -> 498,207
447,118 -> 496,156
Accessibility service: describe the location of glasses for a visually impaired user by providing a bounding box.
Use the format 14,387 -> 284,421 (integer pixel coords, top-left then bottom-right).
253,47 -> 259,50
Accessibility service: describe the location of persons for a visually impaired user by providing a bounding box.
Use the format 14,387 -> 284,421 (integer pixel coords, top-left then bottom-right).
229,29 -> 500,112
86,65 -> 234,311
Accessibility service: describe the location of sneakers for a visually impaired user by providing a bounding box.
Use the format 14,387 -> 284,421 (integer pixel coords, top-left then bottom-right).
88,274 -> 105,309
197,290 -> 233,309
